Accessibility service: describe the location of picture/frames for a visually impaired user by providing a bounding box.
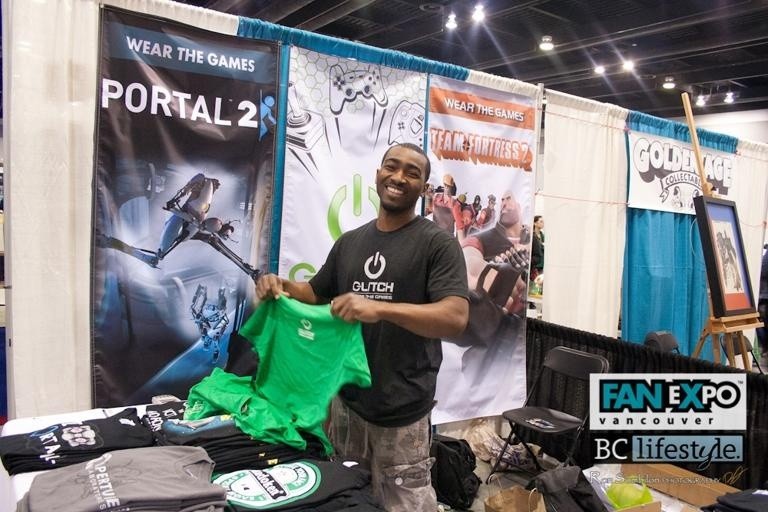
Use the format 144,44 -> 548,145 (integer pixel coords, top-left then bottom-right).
692,195 -> 756,318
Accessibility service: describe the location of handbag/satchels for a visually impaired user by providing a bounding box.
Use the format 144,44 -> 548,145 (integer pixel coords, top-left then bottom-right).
484,484 -> 546,512
533,466 -> 584,512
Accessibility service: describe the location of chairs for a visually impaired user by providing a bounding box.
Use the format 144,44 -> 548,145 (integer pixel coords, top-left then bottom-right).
645,330 -> 764,375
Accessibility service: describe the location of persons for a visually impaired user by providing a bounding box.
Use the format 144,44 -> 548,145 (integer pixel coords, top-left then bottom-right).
757,244 -> 768,359
252,141 -> 470,511
527,215 -> 544,310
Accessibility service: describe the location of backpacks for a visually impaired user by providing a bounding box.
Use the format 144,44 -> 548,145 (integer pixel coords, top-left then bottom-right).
429,433 -> 483,512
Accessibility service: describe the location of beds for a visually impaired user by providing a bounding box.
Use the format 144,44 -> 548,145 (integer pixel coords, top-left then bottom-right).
481,345 -> 610,494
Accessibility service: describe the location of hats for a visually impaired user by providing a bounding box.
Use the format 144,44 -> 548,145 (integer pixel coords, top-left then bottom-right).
443,173 -> 454,187
